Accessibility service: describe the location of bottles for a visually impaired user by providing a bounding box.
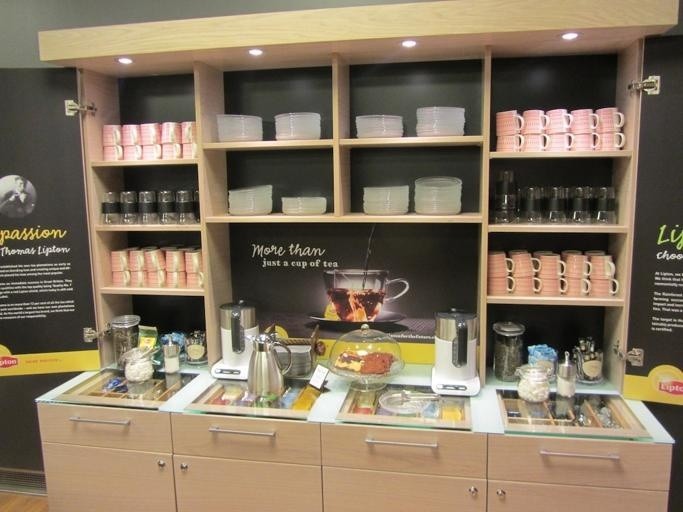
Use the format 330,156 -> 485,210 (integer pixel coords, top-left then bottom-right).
111,313 -> 140,366
162,336 -> 179,374
491,319 -> 525,383
124,357 -> 152,381
556,349 -> 577,398
513,362 -> 552,404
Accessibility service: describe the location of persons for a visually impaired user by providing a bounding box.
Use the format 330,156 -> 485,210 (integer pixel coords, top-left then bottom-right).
1,177 -> 32,216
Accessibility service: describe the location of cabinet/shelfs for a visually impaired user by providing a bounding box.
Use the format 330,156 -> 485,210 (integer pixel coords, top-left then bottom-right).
319,421 -> 673,510
35,401 -> 323,512
187,44 -> 484,223
75,35 -> 227,372
476,35 -> 646,394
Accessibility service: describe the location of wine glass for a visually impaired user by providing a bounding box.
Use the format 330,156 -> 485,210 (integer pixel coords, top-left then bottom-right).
325,357 -> 405,392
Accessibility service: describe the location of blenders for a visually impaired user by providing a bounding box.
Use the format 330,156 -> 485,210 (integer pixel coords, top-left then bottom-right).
430,307 -> 483,396
211,298 -> 259,380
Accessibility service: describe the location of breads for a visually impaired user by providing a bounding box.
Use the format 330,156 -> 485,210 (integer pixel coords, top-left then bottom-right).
334,350 -> 395,375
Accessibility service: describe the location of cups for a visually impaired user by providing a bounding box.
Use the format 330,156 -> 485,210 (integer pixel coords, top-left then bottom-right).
487,250 -> 620,297
100,121 -> 197,159
494,168 -> 617,225
100,189 -> 201,224
321,269 -> 410,319
183,337 -> 207,364
494,107 -> 626,151
111,244 -> 204,288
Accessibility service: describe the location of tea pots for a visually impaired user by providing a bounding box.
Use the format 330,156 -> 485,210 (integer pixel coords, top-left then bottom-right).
243,332 -> 292,396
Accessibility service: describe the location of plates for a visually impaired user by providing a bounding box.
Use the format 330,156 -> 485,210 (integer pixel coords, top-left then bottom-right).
354,113 -> 405,138
413,175 -> 464,216
413,107 -> 468,138
279,195 -> 327,215
214,114 -> 265,142
361,185 -> 411,215
272,112 -> 323,141
308,309 -> 407,326
226,183 -> 274,215
